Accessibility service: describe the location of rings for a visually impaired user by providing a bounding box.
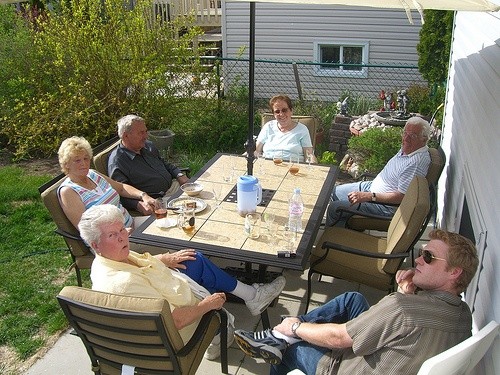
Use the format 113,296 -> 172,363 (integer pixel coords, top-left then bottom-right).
181,253 -> 184,256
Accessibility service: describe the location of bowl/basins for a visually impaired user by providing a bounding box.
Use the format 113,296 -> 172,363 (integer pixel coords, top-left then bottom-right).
180,182 -> 204,195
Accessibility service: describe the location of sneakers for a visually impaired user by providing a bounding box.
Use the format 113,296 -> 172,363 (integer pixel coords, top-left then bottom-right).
245,275 -> 287,317
233,328 -> 290,365
204,325 -> 234,360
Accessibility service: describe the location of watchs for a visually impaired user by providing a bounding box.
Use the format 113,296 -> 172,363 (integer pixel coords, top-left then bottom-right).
370,193 -> 377,203
176,172 -> 184,178
292,321 -> 302,339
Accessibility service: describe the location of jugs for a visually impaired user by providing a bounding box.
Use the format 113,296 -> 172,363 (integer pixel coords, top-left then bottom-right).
237,175 -> 263,217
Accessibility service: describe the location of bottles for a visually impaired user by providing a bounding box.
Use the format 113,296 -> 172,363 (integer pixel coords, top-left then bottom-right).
288,188 -> 304,232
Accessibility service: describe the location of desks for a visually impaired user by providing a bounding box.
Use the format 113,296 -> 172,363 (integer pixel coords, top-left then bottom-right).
128,153 -> 340,331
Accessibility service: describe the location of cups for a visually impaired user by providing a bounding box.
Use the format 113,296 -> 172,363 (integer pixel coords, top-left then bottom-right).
154,198 -> 167,219
273,152 -> 283,166
289,157 -> 300,175
177,208 -> 195,234
245,213 -> 261,239
223,172 -> 233,184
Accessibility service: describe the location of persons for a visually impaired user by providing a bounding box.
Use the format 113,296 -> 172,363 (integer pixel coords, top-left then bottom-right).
57,135 -> 155,237
109,114 -> 188,215
254,94 -> 316,163
234,230 -> 480,375
77,203 -> 287,360
325,117 -> 431,229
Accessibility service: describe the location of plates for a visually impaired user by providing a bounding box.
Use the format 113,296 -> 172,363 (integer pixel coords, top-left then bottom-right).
168,196 -> 207,213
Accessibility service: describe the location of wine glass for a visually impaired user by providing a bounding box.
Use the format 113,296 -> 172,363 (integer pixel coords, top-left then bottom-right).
305,148 -> 314,169
264,207 -> 297,252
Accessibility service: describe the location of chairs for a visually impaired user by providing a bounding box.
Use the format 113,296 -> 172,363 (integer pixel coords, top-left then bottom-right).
286,321 -> 500,375
91,135 -> 191,197
261,113 -> 316,148
38,173 -> 144,287
304,174 -> 436,315
55,281 -> 228,375
331,147 -> 443,269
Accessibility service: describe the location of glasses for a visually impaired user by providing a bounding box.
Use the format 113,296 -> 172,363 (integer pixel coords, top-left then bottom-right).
401,130 -> 427,139
418,249 -> 448,264
274,107 -> 288,113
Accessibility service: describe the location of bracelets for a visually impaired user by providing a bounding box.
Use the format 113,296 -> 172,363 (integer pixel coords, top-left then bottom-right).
139,191 -> 146,199
